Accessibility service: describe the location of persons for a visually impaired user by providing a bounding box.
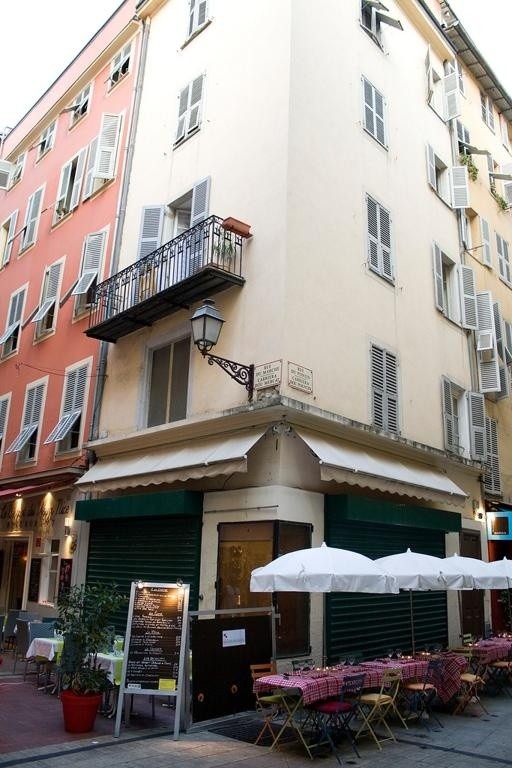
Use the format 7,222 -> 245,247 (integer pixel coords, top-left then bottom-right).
60,559 -> 72,593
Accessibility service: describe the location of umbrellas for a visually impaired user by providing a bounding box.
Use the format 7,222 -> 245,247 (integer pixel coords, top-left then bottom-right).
373,547 -> 474,657
487,556 -> 512,589
249,542 -> 399,671
443,552 -> 509,646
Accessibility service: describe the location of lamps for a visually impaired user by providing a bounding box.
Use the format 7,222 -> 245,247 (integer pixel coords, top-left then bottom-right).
189,298 -> 256,401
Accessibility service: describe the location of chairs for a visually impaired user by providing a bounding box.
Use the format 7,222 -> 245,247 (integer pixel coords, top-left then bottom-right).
0,606 -> 115,699
249,632 -> 511,766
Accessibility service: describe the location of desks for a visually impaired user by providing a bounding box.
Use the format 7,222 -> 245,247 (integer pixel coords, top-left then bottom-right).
90,645 -> 159,719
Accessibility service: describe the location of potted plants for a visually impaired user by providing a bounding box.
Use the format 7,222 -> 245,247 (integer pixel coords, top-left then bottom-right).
53,579 -> 128,733
210,238 -> 233,269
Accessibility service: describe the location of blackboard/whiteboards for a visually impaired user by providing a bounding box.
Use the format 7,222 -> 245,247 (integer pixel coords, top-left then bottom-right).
119,582 -> 191,697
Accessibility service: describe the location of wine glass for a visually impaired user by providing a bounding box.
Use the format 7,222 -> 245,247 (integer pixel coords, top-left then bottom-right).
466,630 -> 512,648
387,649 -> 394,661
395,649 -> 402,661
294,655 -> 356,680
